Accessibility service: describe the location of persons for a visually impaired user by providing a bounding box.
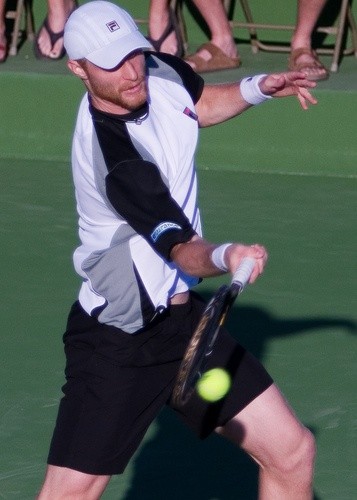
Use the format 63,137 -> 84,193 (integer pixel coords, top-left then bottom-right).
38,2 -> 316,500
0,0 -> 8,61
183,1 -> 330,82
34,1 -> 177,60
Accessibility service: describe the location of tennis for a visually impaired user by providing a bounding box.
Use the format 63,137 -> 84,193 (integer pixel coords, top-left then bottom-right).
193,365 -> 231,405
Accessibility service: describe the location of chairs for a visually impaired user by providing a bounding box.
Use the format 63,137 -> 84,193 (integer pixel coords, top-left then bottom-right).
60,0 -> 189,54
0,0 -> 35,56
208,0 -> 356,71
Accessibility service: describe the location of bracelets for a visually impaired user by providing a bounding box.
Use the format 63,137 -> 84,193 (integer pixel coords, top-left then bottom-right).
240,72 -> 271,105
211,243 -> 236,271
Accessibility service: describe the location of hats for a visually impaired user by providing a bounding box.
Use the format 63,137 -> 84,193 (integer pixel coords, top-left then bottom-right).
64,1 -> 152,70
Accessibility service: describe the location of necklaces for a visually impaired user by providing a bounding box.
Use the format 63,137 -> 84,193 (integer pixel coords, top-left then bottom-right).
107,111 -> 149,125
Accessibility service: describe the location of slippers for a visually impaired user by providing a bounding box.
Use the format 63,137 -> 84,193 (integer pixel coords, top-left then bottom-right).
36,18 -> 65,60
288,47 -> 328,80
149,11 -> 182,57
184,43 -> 240,73
